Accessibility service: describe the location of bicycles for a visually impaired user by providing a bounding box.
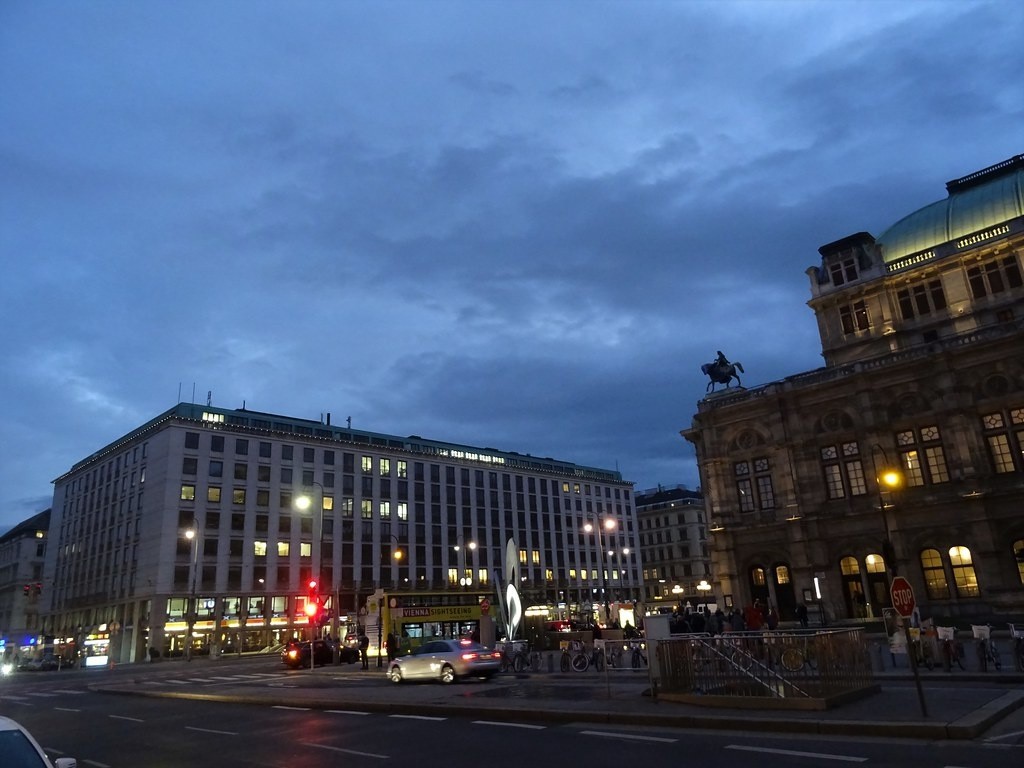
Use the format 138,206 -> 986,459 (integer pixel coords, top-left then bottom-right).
495,613 -> 1024,674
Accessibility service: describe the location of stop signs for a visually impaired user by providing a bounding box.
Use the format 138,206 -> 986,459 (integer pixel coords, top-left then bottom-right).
890,576 -> 916,620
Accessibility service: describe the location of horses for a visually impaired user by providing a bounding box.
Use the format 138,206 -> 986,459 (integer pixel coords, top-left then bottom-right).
701,362 -> 745,393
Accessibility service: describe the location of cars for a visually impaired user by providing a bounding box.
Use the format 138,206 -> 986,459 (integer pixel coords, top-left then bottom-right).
0,647 -> 80,678
0,715 -> 78,768
386,637 -> 502,687
281,639 -> 362,670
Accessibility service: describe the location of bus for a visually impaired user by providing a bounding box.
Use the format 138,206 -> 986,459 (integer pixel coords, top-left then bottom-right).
360,590 -> 504,660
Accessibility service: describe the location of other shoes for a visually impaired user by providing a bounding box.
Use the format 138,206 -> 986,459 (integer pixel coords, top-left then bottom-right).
366,666 -> 368,670
360,666 -> 366,670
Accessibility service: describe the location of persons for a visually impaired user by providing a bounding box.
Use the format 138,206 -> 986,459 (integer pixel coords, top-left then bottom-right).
386,631 -> 411,663
715,351 -> 730,380
358,631 -> 369,670
548,618 -> 622,666
670,601 -> 780,635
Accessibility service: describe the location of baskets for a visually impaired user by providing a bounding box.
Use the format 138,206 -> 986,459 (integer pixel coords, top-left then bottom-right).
1010,624 -> 1024,638
909,628 -> 921,640
572,642 -> 582,651
496,644 -> 505,653
512,644 -> 523,652
972,626 -> 990,639
937,627 -> 954,640
594,639 -> 605,649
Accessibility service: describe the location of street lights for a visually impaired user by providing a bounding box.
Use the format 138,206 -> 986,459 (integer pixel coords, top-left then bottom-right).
377,533 -> 402,666
586,508 -> 617,618
297,481 -> 324,607
182,516 -> 198,661
455,535 -> 478,591
867,440 -> 903,580
